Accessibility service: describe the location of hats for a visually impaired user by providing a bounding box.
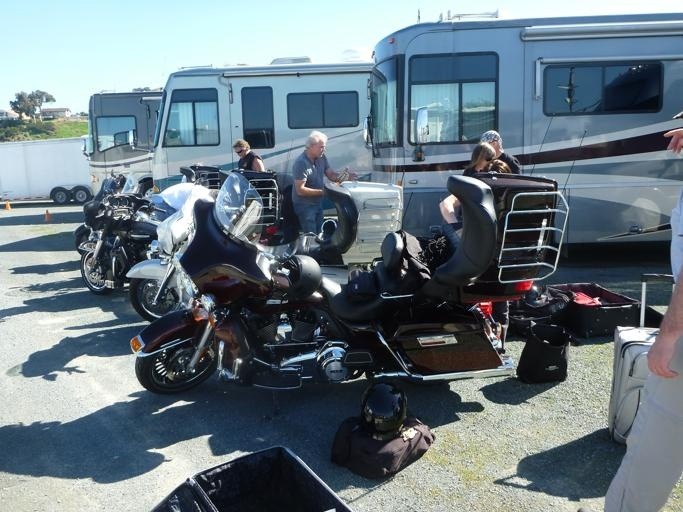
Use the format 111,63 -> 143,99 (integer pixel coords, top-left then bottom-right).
481,131 -> 501,144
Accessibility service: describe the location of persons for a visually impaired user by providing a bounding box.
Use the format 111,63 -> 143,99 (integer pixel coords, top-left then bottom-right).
438,128 -> 520,224
233,138 -> 264,172
292,130 -> 355,233
578,129 -> 683,511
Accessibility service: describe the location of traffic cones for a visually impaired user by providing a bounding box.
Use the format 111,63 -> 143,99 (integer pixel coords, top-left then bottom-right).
4,200 -> 12,211
44,208 -> 52,223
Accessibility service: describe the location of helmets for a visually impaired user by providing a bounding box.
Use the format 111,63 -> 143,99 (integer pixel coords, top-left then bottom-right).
272,255 -> 321,300
361,382 -> 407,441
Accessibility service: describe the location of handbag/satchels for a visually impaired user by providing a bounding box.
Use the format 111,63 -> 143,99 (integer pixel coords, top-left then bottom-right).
517,326 -> 570,384
348,268 -> 379,300
330,415 -> 435,478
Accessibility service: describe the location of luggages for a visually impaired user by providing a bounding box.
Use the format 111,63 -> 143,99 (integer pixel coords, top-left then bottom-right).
608,274 -> 676,443
546,282 -> 665,339
150,448 -> 352,512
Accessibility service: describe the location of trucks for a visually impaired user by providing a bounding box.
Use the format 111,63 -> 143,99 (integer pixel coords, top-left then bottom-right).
0,137 -> 95,205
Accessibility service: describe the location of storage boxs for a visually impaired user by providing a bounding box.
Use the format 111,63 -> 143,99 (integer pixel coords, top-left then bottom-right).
545,281 -> 642,340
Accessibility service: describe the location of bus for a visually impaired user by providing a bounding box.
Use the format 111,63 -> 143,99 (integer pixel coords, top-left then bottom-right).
145,56 -> 377,188
79,89 -> 163,198
363,6 -> 683,262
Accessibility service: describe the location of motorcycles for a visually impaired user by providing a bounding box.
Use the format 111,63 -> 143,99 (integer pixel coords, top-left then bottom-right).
70,165 -> 566,404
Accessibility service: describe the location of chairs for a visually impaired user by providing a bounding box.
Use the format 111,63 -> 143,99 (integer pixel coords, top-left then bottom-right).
319,233 -> 405,321
